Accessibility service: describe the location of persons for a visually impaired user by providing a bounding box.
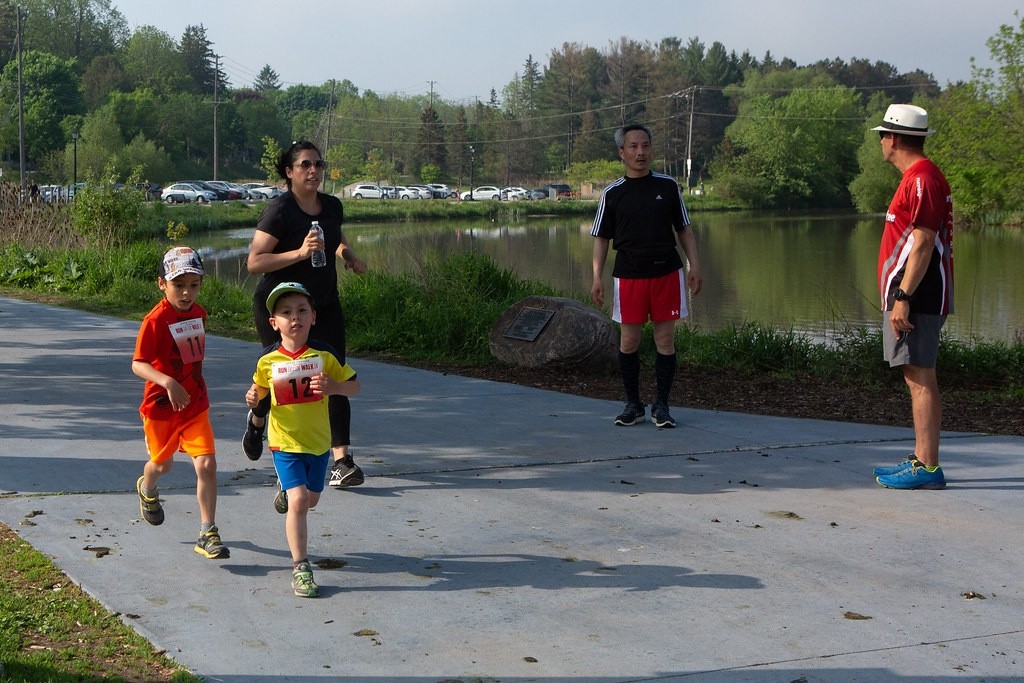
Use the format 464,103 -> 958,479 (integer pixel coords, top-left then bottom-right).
241,142 -> 367,489
589,125 -> 703,428
871,103 -> 955,490
245,282 -> 361,597
131,247 -> 230,559
30,180 -> 38,203
144,179 -> 151,201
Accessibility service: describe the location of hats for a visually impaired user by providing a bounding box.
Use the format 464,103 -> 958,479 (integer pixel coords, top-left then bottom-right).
266,282 -> 310,316
870,104 -> 936,135
160,246 -> 204,281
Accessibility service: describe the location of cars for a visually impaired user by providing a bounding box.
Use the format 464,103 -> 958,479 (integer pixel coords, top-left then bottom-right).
38,182 -> 164,202
506,184 -> 570,202
405,184 -> 453,200
380,186 -> 399,200
395,185 -> 420,200
159,182 -> 219,203
460,186 -> 508,202
176,179 -> 280,199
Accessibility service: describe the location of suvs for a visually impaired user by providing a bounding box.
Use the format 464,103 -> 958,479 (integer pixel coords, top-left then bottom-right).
352,185 -> 390,199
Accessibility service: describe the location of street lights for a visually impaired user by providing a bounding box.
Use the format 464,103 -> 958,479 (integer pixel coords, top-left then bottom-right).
73,128 -> 79,205
468,145 -> 476,202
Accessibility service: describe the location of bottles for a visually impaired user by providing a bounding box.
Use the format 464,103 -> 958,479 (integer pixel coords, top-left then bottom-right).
309,220 -> 326,267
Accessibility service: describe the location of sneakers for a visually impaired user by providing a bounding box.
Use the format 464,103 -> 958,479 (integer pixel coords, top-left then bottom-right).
275,480 -> 289,515
137,476 -> 165,526
615,403 -> 646,426
242,410 -> 267,461
873,453 -> 916,478
651,403 -> 677,427
329,452 -> 365,486
193,525 -> 230,558
876,461 -> 946,490
291,563 -> 320,597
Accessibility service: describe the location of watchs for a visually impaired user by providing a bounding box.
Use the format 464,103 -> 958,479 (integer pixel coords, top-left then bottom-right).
893,289 -> 911,301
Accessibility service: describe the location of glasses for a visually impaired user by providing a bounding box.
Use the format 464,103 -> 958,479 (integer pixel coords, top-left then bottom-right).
293,161 -> 325,169
